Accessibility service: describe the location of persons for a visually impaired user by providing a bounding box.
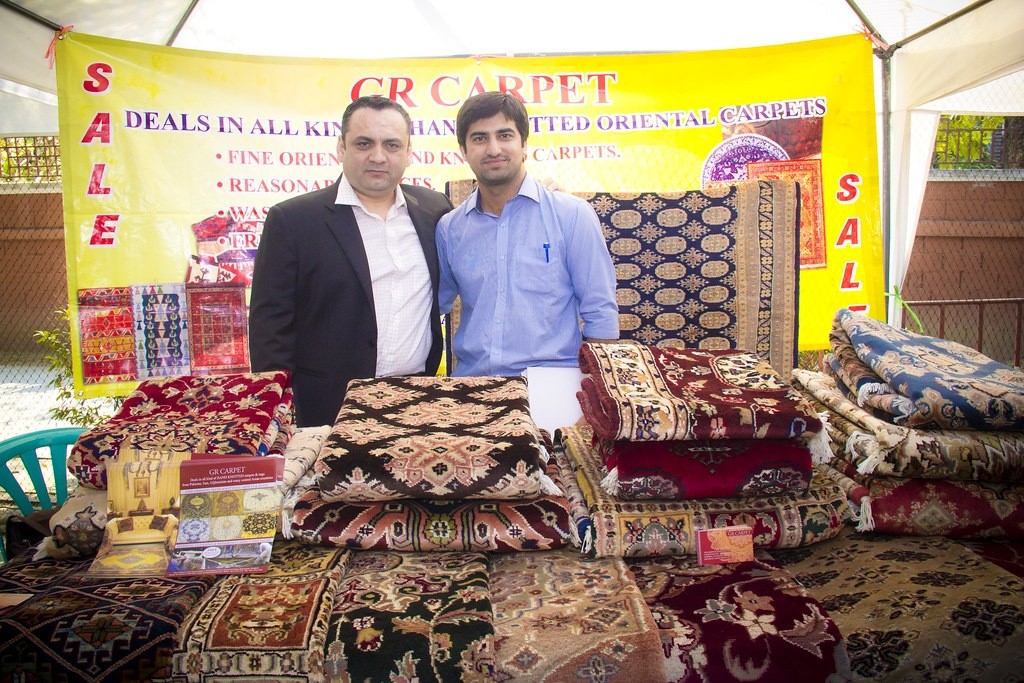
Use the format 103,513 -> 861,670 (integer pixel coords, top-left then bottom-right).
248,96 -> 455,428
435,92 -> 621,377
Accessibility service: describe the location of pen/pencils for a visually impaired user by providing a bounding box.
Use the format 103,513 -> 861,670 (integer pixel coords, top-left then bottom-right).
543,242 -> 550,264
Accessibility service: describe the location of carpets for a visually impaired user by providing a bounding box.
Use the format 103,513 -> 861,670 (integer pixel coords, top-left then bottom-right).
701,114 -> 828,273
0,311 -> 1021,682
446,178 -> 801,380
75,215 -> 259,384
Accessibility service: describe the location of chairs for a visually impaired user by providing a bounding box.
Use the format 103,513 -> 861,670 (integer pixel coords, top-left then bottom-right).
0,427 -> 95,565
